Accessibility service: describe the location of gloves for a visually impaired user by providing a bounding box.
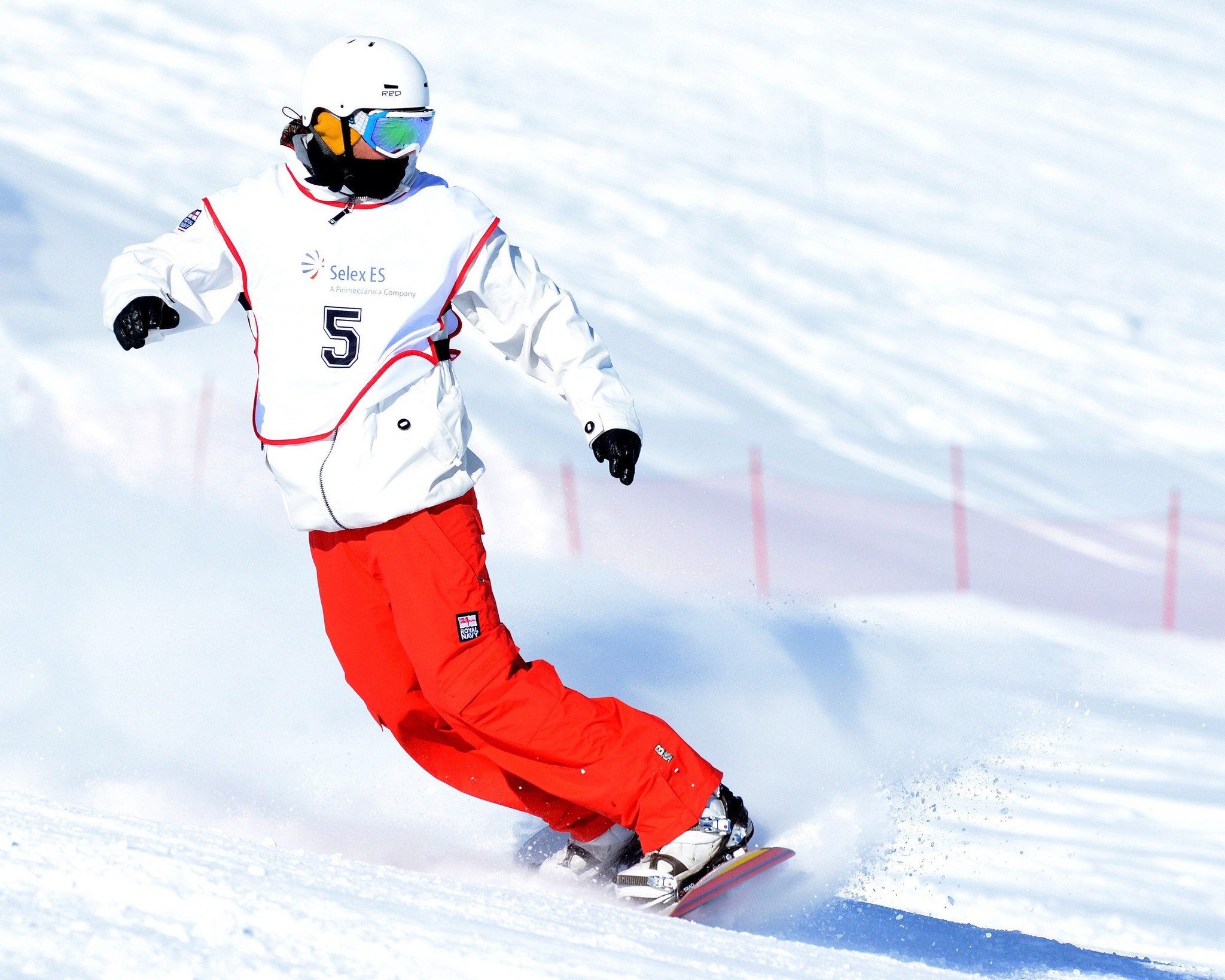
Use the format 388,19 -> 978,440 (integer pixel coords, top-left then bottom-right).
593,428 -> 642,485
114,296 -> 180,351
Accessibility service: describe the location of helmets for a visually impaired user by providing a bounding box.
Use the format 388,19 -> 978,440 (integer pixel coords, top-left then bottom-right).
302,36 -> 429,125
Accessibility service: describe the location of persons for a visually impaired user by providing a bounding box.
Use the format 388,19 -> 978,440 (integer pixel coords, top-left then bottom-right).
101,37 -> 756,904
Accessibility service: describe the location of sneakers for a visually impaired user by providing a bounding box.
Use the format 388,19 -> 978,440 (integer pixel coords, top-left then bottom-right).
537,823 -> 634,881
617,795 -> 753,899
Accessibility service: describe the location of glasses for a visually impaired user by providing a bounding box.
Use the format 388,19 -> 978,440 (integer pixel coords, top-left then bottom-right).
362,110 -> 433,158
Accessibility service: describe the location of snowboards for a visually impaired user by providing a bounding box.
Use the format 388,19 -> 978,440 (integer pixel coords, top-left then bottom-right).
512,821 -> 795,918
